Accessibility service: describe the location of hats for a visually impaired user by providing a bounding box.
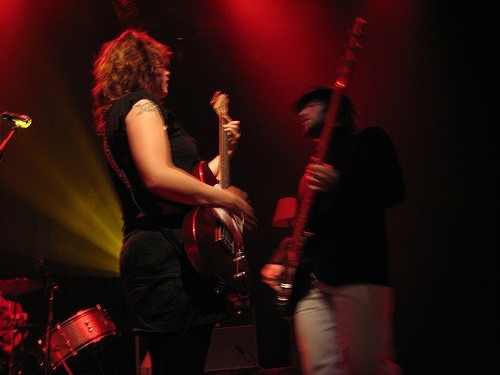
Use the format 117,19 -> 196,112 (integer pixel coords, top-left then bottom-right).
294,88 -> 356,114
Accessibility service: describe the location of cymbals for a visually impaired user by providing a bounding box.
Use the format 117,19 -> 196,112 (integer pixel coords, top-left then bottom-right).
0,275 -> 42,294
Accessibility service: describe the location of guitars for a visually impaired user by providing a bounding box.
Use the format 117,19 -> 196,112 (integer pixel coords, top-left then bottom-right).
186,92 -> 254,275
266,16 -> 369,304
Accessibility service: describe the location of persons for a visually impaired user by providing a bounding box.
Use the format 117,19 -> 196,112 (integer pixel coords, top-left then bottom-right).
94,29 -> 261,375
261,86 -> 408,375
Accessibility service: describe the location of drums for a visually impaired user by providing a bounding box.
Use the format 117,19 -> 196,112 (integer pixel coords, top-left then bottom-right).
39,305 -> 121,371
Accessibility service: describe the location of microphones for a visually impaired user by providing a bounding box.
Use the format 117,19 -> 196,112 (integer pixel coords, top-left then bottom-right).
2,112 -> 32,128
